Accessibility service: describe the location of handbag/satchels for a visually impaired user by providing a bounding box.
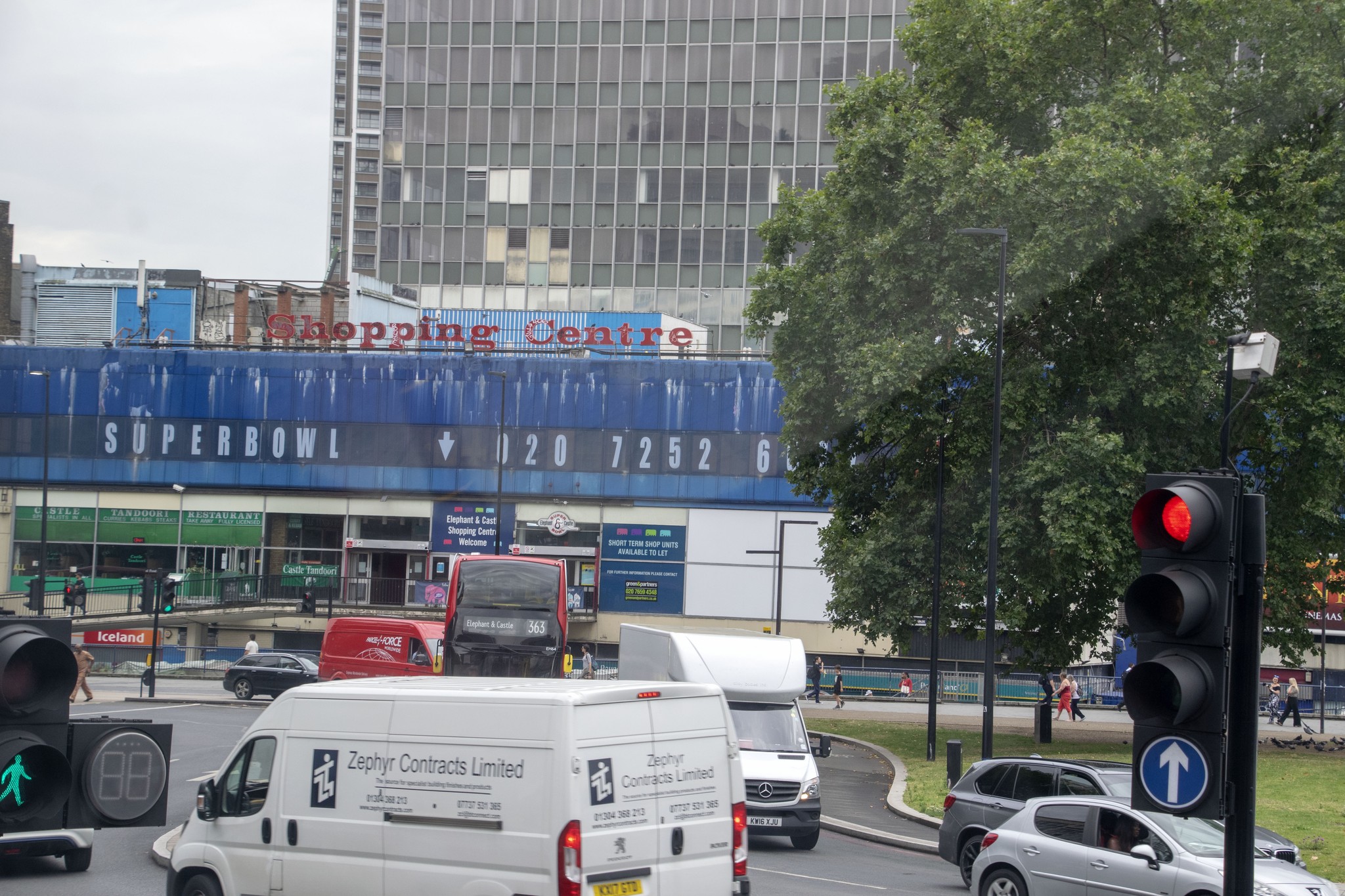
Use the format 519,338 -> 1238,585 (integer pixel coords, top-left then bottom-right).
1058,693 -> 1061,699
901,685 -> 910,694
586,653 -> 598,671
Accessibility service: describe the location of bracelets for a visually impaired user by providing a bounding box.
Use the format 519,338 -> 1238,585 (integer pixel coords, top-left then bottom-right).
1272,689 -> 1274,692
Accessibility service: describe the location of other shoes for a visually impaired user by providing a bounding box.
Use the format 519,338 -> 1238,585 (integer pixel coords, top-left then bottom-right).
84,698 -> 93,702
69,698 -> 74,702
1117,704 -> 1121,712
1267,721 -> 1274,724
816,701 -> 823,704
1052,711 -> 1055,713
806,695 -> 809,702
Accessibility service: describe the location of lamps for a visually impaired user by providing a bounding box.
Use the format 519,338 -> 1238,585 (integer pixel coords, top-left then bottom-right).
484,352 -> 491,357
320,346 -> 326,352
463,341 -> 476,357
100,340 -> 114,348
857,648 -> 864,654
272,624 -> 278,628
1001,653 -> 1008,662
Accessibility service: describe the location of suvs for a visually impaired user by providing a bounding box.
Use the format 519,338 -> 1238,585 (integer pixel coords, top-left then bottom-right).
938,757 -> 1307,889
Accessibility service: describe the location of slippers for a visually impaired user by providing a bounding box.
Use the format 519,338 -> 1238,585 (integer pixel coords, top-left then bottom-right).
1275,722 -> 1282,726
1294,725 -> 1301,727
1073,720 -> 1076,722
1080,716 -> 1086,722
1065,719 -> 1073,722
1052,717 -> 1059,721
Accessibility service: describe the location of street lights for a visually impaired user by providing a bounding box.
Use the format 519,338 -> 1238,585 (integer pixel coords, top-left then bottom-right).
962,228 -> 1007,759
29,370 -> 51,615
747,520 -> 818,633
488,371 -> 505,556
173,485 -> 186,575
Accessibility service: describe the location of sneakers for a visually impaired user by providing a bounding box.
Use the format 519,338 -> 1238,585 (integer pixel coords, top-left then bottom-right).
840,701 -> 845,708
833,705 -> 840,709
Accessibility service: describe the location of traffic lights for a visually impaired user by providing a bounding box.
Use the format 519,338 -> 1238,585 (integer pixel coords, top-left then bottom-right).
161,579 -> 175,614
137,577 -> 154,614
0,614 -> 174,834
63,585 -> 86,606
1118,472 -> 1241,819
296,591 -> 314,613
23,579 -> 39,610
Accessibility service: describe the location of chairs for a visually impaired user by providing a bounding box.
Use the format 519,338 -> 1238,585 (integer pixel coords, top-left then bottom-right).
1101,812 -> 1117,830
1137,826 -> 1149,846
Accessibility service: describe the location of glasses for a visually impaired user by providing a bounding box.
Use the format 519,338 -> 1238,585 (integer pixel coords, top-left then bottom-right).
1274,675 -> 1279,679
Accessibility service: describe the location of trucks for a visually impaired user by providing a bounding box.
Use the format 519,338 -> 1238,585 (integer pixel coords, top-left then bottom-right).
316,617 -> 445,683
618,623 -> 832,849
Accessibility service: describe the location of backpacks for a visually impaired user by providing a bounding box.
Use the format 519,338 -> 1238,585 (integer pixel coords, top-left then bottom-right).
807,667 -> 817,680
1038,673 -> 1048,685
1072,681 -> 1083,696
1122,670 -> 1128,685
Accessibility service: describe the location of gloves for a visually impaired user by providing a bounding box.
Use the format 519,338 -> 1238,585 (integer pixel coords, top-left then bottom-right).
1262,682 -> 1266,686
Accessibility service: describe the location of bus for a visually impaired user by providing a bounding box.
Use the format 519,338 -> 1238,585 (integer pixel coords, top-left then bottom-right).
432,555 -> 573,679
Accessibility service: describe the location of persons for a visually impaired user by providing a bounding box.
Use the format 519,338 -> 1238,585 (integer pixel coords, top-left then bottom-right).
898,671 -> 912,698
864,690 -> 873,696
832,664 -> 846,710
284,659 -> 296,670
66,572 -> 89,617
1038,661 -> 1056,713
412,643 -> 430,665
1051,672 -> 1073,722
1275,678 -> 1302,727
805,656 -> 824,704
1117,662 -> 1135,712
243,633 -> 259,656
1066,674 -> 1086,722
69,644 -> 95,703
1262,674 -> 1282,725
579,644 -> 595,680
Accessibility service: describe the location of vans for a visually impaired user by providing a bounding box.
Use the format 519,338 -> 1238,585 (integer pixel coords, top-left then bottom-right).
168,674 -> 752,896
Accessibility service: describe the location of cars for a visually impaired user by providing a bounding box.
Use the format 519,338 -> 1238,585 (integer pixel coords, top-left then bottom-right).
969,796 -> 1340,896
224,653 -> 321,700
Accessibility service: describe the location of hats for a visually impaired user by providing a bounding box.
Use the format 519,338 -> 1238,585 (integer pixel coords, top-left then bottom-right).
75,572 -> 82,576
865,690 -> 872,695
